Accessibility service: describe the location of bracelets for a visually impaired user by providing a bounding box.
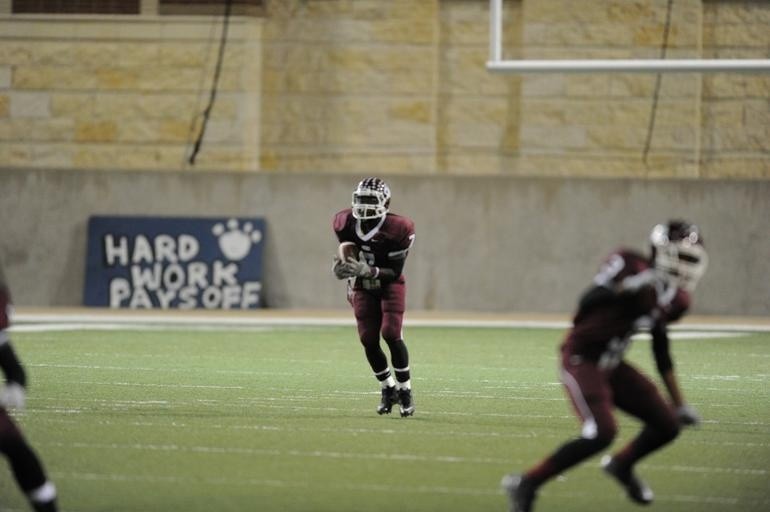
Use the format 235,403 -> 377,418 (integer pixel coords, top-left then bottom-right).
370,267 -> 379,279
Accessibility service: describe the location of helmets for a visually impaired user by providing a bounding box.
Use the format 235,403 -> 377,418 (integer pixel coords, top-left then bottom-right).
650,220 -> 707,262
352,176 -> 391,210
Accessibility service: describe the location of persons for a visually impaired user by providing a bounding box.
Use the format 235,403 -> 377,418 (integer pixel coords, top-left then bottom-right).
0,277 -> 60,511
327,175 -> 417,420
496,217 -> 709,512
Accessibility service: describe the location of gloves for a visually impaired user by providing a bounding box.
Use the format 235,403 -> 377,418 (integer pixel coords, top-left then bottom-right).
332,251 -> 379,280
676,410 -> 699,427
623,269 -> 668,294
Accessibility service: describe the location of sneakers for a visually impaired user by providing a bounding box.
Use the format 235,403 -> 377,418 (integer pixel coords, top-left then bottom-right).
502,476 -> 535,512
601,456 -> 652,504
377,384 -> 415,417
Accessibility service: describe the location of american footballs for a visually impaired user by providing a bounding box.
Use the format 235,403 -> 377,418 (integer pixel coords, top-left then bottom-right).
338,238 -> 359,264
